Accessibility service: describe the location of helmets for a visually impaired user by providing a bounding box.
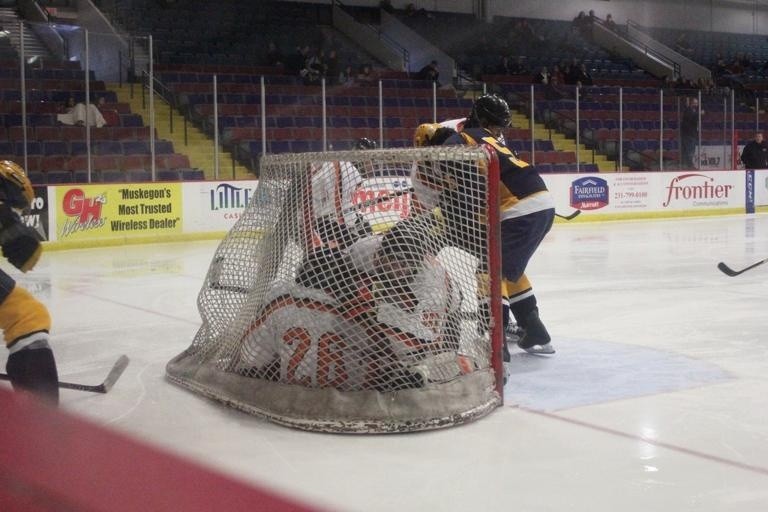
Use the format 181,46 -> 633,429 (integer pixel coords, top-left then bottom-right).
381,221 -> 429,268
468,93 -> 513,127
295,246 -> 354,300
412,122 -> 443,148
348,236 -> 383,276
355,137 -> 376,162
0,159 -> 48,275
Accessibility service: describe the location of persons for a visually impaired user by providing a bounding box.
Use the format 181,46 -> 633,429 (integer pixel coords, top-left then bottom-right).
268,39 -> 381,85
682,98 -> 704,167
571,63 -> 593,100
508,9 -> 619,56
740,131 -> 768,168
568,57 -> 580,82
407,3 -> 425,16
64,96 -> 76,112
413,60 -> 443,88
536,65 -> 551,84
409,98 -> 556,384
231,141 -> 482,387
551,64 -> 567,86
0,156 -> 61,404
96,96 -> 106,109
670,31 -> 768,80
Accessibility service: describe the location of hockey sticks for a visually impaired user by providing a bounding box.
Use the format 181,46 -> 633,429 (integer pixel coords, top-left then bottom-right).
0,355 -> 129,394
210,258 -> 251,293
718,259 -> 767,276
555,210 -> 581,220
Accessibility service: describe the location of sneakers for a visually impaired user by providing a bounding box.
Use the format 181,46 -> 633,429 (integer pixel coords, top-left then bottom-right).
505,318 -> 518,339
517,318 -> 551,348
